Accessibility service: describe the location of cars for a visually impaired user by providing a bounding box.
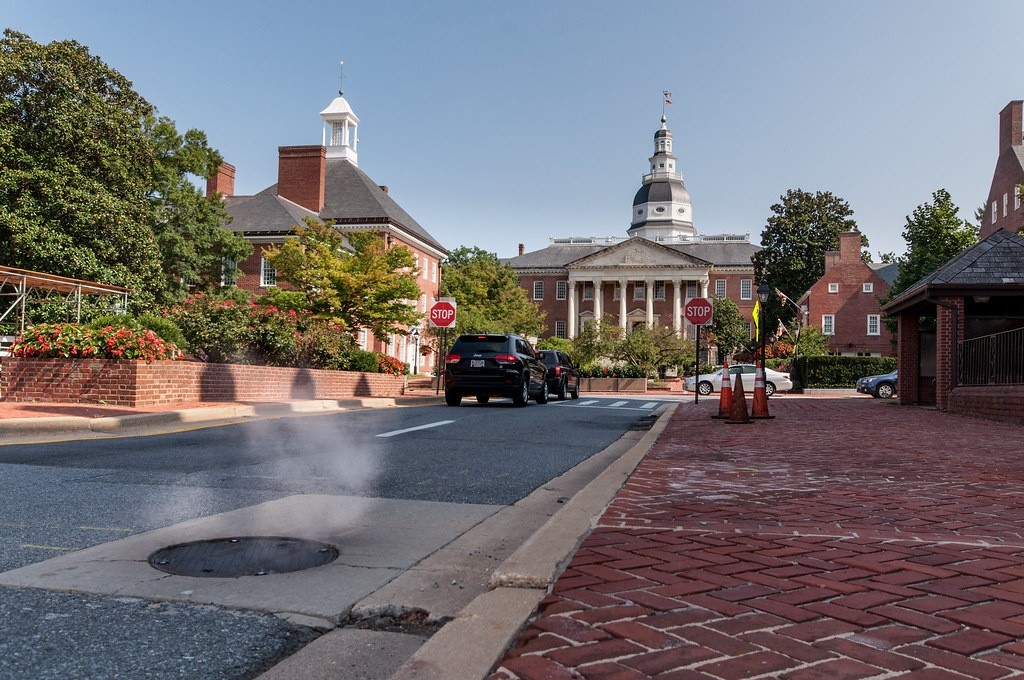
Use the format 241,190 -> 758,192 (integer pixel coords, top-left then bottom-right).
857,370 -> 898,399
682,364 -> 793,395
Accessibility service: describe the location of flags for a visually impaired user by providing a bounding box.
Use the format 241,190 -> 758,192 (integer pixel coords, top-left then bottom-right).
665,99 -> 672,104
663,93 -> 672,98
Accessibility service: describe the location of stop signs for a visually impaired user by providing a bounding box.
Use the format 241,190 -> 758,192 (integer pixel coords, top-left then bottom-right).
683,298 -> 713,326
428,301 -> 457,328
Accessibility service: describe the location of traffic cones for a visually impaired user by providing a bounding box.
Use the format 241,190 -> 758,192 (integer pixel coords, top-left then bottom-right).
710,360 -> 734,419
722,372 -> 754,424
748,360 -> 775,419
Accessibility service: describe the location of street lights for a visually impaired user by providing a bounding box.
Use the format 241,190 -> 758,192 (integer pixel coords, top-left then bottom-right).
756,278 -> 770,397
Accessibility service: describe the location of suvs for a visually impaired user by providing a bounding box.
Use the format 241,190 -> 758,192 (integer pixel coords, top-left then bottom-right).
538,350 -> 580,400
445,330 -> 549,407
